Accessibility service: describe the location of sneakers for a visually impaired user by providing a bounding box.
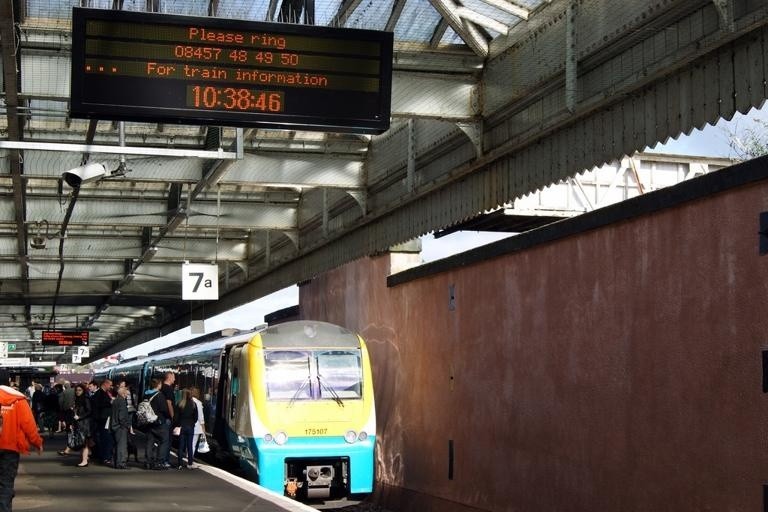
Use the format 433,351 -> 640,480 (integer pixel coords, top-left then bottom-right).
144,463 -> 175,470
79,462 -> 89,467
177,464 -> 198,470
57,450 -> 69,456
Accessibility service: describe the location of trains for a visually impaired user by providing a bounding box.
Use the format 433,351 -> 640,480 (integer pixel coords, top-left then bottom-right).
89,320 -> 381,510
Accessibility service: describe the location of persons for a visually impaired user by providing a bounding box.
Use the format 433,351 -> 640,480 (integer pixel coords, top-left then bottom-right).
0,368 -> 219,473
0,368 -> 43,512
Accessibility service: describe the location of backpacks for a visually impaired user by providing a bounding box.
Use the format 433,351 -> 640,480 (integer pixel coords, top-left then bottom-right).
137,393 -> 159,426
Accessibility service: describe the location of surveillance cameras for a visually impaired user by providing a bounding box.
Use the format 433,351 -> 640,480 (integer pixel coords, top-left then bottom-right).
30,237 -> 47,249
62,162 -> 106,187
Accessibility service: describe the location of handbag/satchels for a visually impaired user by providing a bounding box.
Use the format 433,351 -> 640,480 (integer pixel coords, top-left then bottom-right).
173,427 -> 181,436
198,434 -> 210,453
